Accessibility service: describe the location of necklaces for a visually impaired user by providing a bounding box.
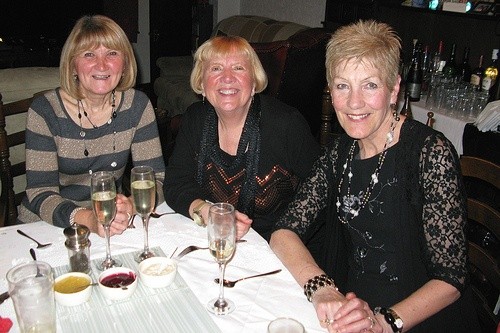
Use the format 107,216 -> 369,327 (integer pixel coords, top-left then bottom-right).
335,112 -> 400,224
78,89 -> 117,176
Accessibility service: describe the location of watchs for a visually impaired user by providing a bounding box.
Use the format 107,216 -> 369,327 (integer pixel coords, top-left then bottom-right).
388,309 -> 404,333
192,202 -> 207,228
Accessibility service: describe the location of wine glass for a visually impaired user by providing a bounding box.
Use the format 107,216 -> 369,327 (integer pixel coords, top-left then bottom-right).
207,203 -> 238,315
89,171 -> 122,271
130,165 -> 160,263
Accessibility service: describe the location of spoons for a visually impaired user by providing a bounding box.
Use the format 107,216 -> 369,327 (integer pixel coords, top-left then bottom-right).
150,212 -> 178,218
214,269 -> 282,287
17,229 -> 52,249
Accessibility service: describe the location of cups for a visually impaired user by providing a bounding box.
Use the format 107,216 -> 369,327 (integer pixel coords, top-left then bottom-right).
6,262 -> 57,333
267,317 -> 305,333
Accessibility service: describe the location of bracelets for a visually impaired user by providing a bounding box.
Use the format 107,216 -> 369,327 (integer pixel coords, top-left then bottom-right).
303,274 -> 338,303
376,307 -> 398,333
69,207 -> 86,226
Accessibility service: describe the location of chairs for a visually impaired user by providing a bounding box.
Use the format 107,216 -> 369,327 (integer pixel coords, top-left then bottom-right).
443,154 -> 500,326
0,94 -> 34,227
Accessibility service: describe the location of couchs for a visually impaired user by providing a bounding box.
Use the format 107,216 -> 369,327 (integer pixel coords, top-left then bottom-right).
151,15 -> 339,128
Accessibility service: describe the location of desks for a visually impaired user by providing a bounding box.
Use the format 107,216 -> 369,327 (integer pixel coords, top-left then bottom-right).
395,82 -> 494,159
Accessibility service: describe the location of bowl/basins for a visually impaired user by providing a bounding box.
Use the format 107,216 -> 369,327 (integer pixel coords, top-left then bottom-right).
97,267 -> 139,301
53,272 -> 92,307
138,256 -> 178,287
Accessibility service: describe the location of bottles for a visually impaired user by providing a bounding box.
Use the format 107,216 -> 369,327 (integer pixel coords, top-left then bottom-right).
64,222 -> 93,275
400,39 -> 499,119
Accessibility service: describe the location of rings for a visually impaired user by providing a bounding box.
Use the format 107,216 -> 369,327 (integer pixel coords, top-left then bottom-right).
368,317 -> 374,329
320,320 -> 327,322
369,330 -> 374,333
327,319 -> 335,324
127,212 -> 131,220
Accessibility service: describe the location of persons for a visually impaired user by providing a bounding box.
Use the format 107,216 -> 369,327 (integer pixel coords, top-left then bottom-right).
162,35 -> 314,243
16,15 -> 166,238
267,19 -> 477,333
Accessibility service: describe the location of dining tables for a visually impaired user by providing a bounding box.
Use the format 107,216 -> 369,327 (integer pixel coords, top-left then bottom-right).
1,202 -> 328,333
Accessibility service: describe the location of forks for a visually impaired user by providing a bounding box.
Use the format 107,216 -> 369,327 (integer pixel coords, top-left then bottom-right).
177,239 -> 247,259
